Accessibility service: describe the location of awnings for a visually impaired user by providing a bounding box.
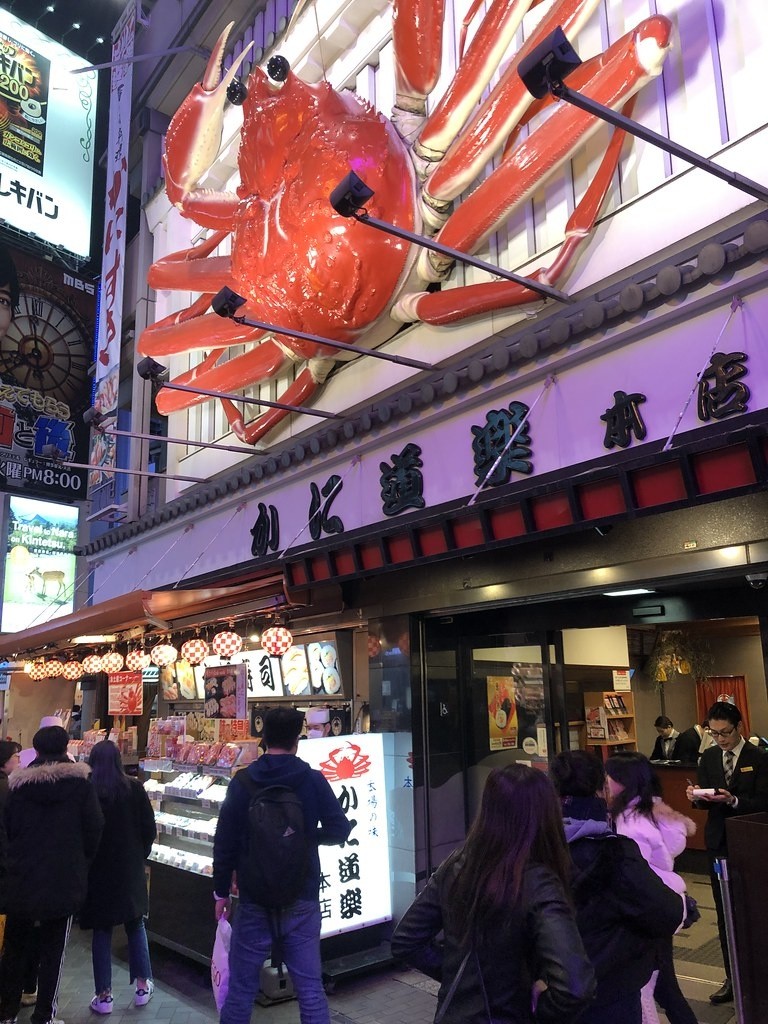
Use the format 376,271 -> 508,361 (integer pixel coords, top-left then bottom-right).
0,454 -> 364,661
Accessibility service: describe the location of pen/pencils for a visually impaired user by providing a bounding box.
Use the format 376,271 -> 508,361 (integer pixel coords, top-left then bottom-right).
687,779 -> 694,787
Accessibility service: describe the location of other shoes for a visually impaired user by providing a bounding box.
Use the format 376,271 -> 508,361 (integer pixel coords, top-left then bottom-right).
21,986 -> 38,1005
52,1018 -> 64,1024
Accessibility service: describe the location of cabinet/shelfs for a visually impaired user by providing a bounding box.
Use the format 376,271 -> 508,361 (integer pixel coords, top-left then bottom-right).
585,691 -> 638,767
140,757 -> 258,968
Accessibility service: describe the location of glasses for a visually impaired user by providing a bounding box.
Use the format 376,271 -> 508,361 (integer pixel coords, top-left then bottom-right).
711,726 -> 735,737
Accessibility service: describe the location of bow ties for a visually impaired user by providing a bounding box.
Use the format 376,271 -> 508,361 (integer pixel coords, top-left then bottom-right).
664,737 -> 673,742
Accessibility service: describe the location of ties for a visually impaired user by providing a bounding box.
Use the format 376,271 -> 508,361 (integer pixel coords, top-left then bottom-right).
724,751 -> 735,786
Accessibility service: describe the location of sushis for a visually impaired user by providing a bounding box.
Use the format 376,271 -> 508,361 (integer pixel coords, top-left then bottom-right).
205,675 -> 237,718
280,642 -> 340,695
496,698 -> 512,729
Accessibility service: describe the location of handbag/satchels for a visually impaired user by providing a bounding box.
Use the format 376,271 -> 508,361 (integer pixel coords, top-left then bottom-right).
210,913 -> 233,1015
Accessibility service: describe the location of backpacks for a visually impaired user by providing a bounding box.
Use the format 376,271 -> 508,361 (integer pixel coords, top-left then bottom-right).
236,768 -> 311,909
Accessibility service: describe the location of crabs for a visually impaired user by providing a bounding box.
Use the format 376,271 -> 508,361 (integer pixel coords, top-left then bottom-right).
138,0 -> 671,445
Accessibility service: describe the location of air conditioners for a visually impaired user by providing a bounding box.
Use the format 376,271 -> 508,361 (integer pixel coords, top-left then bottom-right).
328,170 -> 571,302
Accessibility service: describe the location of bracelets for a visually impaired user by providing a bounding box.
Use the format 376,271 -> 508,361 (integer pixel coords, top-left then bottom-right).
727,797 -> 735,806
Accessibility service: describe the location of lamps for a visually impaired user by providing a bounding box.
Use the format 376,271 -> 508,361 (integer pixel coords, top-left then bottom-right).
69,635 -> 122,643
43,444 -> 205,483
211,285 -> 431,370
137,355 -> 334,419
83,406 -> 260,455
516,25 -> 768,205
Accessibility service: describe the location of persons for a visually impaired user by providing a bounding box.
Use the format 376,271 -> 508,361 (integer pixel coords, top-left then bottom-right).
211,709 -> 352,1024
548,751 -> 683,1024
306,707 -> 331,739
650,716 -> 680,760
671,720 -> 710,761
80,740 -> 156,1014
0,727 -> 108,1023
391,763 -> 596,1024
604,751 -> 699,1024
685,702 -> 768,1003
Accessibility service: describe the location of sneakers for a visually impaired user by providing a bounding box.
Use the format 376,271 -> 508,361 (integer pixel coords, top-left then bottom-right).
90,994 -> 113,1013
135,979 -> 154,1005
